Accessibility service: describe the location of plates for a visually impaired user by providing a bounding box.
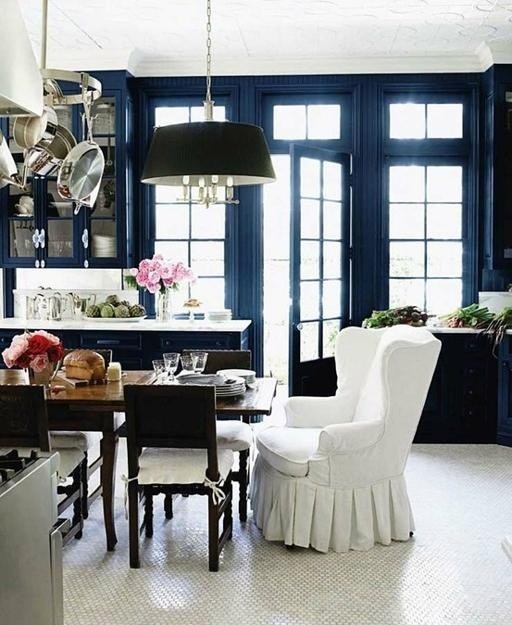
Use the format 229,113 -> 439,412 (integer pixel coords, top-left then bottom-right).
176,367 -> 257,397
81,313 -> 148,322
208,309 -> 233,322
92,233 -> 116,257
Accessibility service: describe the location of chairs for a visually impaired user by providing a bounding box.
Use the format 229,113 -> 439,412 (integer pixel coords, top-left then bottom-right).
163,349 -> 253,521
120,383 -> 233,574
242,323 -> 443,550
0,385 -> 86,547
47,348 -> 112,527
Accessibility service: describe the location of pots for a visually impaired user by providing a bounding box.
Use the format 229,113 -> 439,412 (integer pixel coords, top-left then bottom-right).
1,107 -> 105,214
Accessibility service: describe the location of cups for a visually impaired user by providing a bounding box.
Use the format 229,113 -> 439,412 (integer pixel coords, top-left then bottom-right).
12,236 -> 75,257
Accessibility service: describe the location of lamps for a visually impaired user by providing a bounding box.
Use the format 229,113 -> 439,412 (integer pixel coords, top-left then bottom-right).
138,0 -> 282,207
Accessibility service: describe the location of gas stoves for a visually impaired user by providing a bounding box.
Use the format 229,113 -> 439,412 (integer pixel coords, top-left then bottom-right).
0,444 -> 58,498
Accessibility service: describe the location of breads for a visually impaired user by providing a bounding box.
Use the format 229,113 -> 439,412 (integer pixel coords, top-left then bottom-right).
63,351 -> 105,380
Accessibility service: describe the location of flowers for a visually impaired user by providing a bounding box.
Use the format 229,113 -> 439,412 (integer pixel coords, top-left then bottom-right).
133,254 -> 196,293
1,330 -> 63,372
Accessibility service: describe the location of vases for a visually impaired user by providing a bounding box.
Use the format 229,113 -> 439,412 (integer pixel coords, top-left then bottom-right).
34,364 -> 50,385
156,291 -> 173,322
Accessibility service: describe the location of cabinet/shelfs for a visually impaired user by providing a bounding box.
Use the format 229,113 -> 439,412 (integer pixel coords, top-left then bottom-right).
414,334 -> 497,444
487,95 -> 512,270
0,70 -> 139,270
0,320 -> 248,371
496,335 -> 512,447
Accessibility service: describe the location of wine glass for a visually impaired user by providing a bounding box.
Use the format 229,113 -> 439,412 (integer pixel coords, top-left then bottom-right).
152,350 -> 210,384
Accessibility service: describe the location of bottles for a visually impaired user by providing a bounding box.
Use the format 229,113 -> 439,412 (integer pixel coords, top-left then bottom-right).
107,362 -> 121,381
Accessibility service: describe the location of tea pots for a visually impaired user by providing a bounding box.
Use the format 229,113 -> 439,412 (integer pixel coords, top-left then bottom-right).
13,292 -> 95,322
